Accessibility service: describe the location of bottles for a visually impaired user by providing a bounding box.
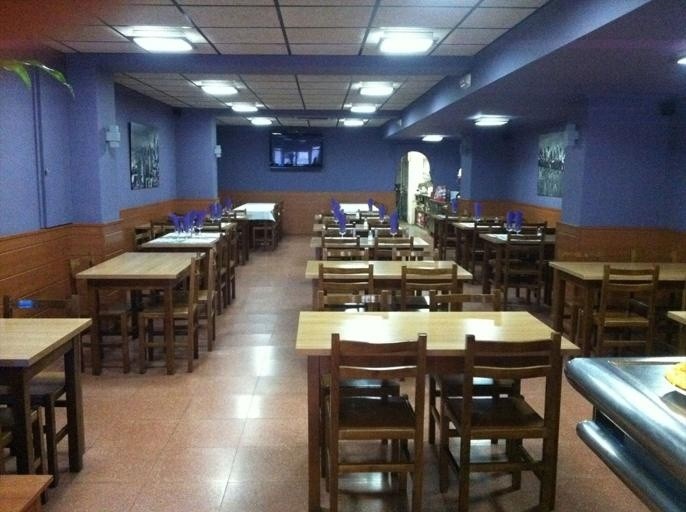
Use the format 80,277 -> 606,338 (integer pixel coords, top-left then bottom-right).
356,210 -> 359,220
368,230 -> 374,242
537,228 -> 541,236
364,219 -> 368,228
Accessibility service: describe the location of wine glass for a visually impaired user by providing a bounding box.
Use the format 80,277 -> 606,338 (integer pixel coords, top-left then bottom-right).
209,216 -> 215,224
334,219 -> 339,225
176,226 -> 182,242
390,232 -> 397,241
195,224 -> 204,237
379,219 -> 384,225
187,226 -> 195,239
514,227 -> 521,234
339,232 -> 345,237
506,225 -> 512,232
473,216 -> 480,223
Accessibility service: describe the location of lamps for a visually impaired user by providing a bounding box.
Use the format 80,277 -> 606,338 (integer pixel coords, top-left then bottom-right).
475,117 -> 509,126
